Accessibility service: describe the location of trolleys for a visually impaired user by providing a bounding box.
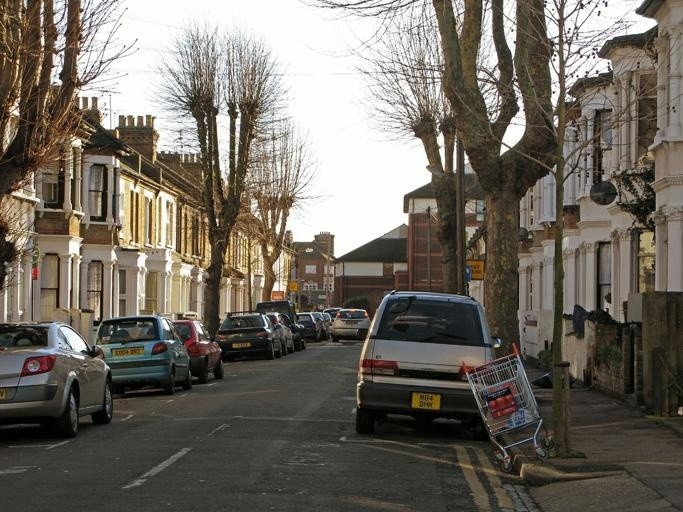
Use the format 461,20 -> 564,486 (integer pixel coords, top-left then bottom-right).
463,343 -> 553,473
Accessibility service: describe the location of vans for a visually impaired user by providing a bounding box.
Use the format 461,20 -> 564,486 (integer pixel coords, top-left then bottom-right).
355,290 -> 501,438
95,315 -> 192,396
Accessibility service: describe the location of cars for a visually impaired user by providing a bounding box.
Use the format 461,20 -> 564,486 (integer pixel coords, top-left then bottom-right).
215,296 -> 373,365
173,319 -> 223,382
0,322 -> 115,440
